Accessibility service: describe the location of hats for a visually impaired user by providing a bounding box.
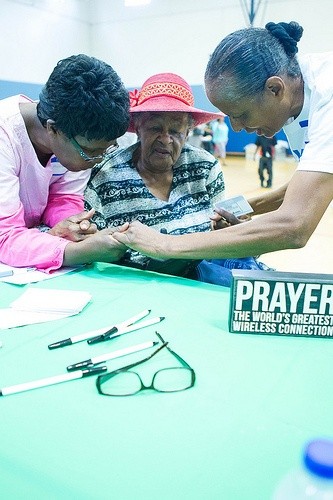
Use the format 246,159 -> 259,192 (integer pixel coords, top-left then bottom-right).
126,73 -> 227,133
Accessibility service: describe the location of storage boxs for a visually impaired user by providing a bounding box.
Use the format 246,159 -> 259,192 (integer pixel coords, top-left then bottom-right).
228,267 -> 333,341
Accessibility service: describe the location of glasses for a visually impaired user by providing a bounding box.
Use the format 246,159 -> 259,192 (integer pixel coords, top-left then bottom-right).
96,331 -> 196,396
58,126 -> 120,163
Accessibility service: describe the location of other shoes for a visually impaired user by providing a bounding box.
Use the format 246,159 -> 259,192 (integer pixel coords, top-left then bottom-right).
261,178 -> 264,186
267,185 -> 272,188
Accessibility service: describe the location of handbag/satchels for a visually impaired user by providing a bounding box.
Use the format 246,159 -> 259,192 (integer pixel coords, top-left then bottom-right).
151,242 -> 276,287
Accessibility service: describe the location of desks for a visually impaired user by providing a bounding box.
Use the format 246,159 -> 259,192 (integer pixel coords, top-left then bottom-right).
0,260 -> 333,500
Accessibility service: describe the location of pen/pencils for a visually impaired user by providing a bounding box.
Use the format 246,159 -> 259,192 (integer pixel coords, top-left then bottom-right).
48,324 -> 114,349
87,316 -> 167,344
100,308 -> 152,338
67,341 -> 158,371
0,366 -> 108,396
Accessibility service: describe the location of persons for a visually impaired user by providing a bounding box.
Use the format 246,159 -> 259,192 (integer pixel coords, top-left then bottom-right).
187,117 -> 229,167
112,21 -> 333,260
252,134 -> 277,188
84,72 -> 275,288
0,54 -> 129,274
